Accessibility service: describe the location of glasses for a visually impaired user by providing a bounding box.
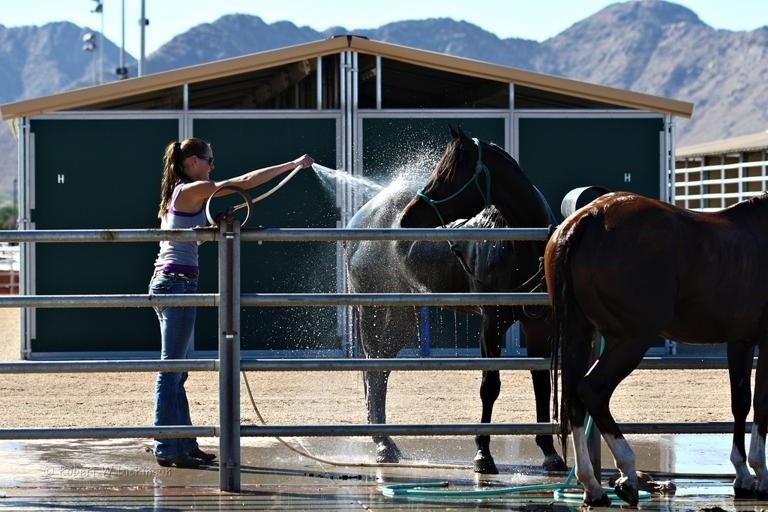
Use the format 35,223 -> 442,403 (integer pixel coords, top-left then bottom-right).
197,155 -> 214,165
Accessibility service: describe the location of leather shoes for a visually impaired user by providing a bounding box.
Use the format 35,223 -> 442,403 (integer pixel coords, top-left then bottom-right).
158,454 -> 204,467
190,448 -> 217,460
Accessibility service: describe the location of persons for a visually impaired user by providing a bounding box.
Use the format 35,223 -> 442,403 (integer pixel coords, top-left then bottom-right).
147,136 -> 313,468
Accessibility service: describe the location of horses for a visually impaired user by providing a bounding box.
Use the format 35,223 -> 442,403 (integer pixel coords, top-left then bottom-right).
343,123 -> 568,474
544,191 -> 768,507
